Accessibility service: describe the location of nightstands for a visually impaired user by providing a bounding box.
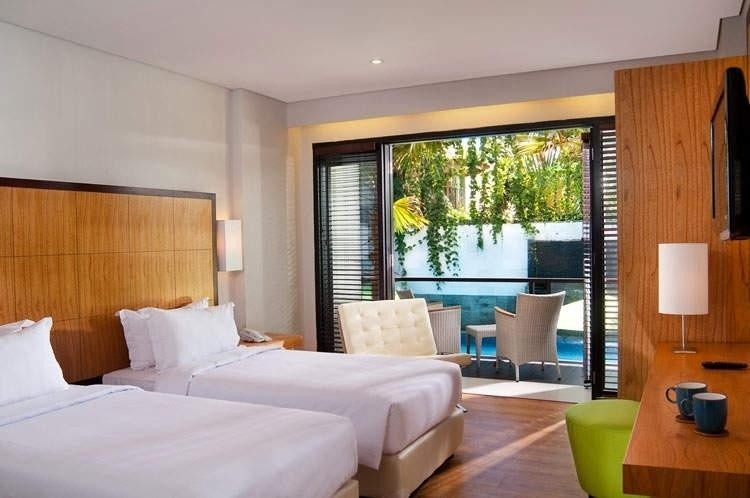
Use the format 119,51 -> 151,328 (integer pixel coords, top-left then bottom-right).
238,330 -> 306,351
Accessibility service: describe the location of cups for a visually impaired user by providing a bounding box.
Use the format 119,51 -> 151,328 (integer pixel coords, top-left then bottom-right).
666,382 -> 706,419
680,392 -> 728,433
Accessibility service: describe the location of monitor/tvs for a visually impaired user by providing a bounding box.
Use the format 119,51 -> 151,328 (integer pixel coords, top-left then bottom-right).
709,67 -> 750,240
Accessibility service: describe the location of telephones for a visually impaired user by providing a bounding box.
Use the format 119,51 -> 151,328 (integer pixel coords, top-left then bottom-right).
238,327 -> 272,343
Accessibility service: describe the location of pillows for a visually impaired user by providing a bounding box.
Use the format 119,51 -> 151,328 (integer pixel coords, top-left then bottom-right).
137,301 -> 241,374
0,313 -> 70,411
113,296 -> 210,371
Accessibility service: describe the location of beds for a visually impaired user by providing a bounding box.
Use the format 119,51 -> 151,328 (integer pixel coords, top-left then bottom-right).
102,344 -> 465,498
0,380 -> 363,498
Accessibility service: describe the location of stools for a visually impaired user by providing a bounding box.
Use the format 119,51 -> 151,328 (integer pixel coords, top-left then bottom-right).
564,399 -> 652,498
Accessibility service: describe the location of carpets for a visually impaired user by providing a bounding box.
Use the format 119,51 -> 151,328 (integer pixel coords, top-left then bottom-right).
460,376 -> 592,404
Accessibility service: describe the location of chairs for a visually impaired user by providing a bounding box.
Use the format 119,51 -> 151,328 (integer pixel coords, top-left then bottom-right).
494,290 -> 567,382
395,287 -> 462,354
336,296 -> 472,413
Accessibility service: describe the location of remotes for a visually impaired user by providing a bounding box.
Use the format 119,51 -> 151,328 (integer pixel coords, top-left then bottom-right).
702,362 -> 748,369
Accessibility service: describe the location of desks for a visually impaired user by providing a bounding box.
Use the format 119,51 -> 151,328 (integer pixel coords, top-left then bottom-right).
621,340 -> 750,498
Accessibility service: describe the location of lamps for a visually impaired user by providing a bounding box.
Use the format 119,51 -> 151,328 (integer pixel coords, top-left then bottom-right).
216,217 -> 243,273
657,242 -> 709,355
